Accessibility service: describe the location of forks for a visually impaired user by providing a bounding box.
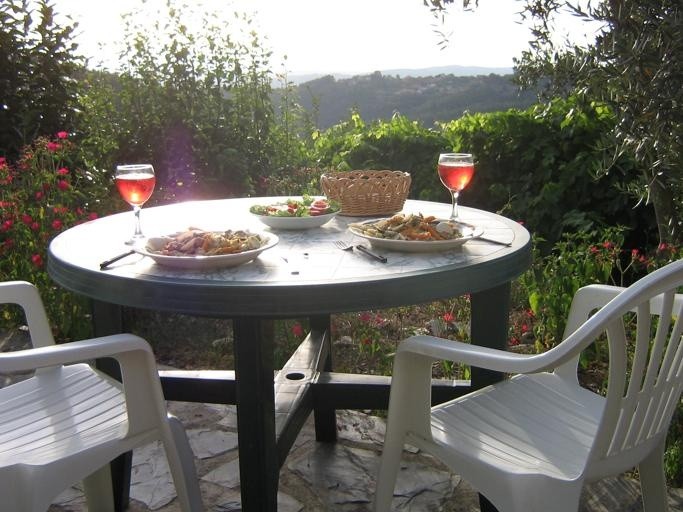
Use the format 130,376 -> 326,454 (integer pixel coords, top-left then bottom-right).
329,239 -> 387,264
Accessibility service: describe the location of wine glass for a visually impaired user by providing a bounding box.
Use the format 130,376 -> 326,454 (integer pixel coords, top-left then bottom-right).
436,152 -> 474,224
113,164 -> 157,248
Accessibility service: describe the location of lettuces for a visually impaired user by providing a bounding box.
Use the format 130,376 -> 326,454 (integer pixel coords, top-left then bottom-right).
250,193 -> 341,217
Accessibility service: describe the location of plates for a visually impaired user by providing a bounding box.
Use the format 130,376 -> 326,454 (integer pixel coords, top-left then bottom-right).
246,205 -> 342,230
128,228 -> 279,268
346,217 -> 482,253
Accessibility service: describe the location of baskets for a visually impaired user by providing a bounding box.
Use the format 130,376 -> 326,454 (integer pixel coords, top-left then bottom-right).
320,170 -> 412,216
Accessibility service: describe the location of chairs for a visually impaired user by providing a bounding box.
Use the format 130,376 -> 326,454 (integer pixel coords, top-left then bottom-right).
0,278 -> 206,511
372,255 -> 682,512
